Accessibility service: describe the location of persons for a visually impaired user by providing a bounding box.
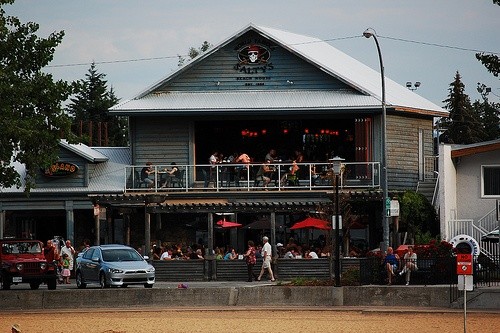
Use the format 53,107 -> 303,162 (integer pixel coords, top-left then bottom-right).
243,240 -> 259,282
209,151 -> 344,186
21,232 -> 90,284
161,162 -> 182,187
136,236 -> 332,259
256,236 -> 276,282
345,244 -> 417,286
141,162 -> 155,188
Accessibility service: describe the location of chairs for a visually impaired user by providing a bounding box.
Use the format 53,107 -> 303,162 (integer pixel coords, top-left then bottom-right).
308,170 -> 346,185
252,167 -> 303,189
135,169 -> 185,192
201,168 -> 241,192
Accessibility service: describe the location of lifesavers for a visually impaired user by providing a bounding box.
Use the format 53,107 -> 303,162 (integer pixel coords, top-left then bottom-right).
448,234 -> 480,262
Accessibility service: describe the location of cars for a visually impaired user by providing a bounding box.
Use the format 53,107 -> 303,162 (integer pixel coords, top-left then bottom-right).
0,238 -> 58,290
74,244 -> 156,289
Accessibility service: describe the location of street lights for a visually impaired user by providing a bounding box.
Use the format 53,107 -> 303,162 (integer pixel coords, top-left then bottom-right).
329,155 -> 346,306
363,27 -> 390,259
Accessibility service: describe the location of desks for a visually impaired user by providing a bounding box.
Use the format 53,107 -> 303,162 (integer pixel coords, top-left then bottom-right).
269,169 -> 286,190
213,171 -> 230,186
154,171 -> 169,188
319,171 -> 329,185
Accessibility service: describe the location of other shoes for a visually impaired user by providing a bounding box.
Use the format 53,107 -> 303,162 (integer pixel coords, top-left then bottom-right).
392,273 -> 396,276
63,281 -> 65,284
268,280 -> 275,282
406,284 -> 409,286
253,279 -> 260,281
245,279 -> 252,282
66,282 -> 71,284
399,272 -> 402,275
387,282 -> 391,286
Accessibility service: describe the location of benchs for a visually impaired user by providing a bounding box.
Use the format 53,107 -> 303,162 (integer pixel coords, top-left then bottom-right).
381,268 -> 432,285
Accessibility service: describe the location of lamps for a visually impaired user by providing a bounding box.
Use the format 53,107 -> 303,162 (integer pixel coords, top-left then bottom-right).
93,203 -> 100,215
333,215 -> 343,229
